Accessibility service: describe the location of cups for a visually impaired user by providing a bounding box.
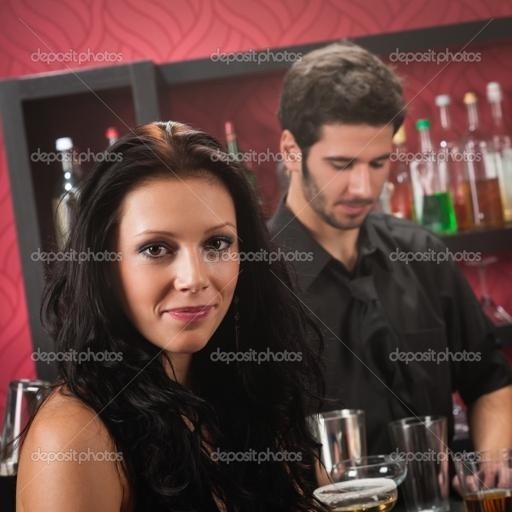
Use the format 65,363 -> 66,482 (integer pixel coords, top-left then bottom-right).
304,408 -> 512,511
1,379 -> 52,477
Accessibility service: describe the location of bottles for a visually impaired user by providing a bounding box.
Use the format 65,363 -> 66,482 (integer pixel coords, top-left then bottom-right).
225,122 -> 263,189
51,136 -> 85,253
388,81 -> 512,235
105,127 -> 118,147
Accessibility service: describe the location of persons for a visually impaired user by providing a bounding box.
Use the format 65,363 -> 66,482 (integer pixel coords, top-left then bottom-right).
268,42 -> 512,503
16,121 -> 325,511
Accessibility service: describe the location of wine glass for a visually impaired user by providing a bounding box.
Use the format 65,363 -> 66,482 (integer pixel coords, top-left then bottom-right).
462,255 -> 511,327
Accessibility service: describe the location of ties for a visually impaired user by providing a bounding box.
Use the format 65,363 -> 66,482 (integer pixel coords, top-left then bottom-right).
324,256 -> 419,418
